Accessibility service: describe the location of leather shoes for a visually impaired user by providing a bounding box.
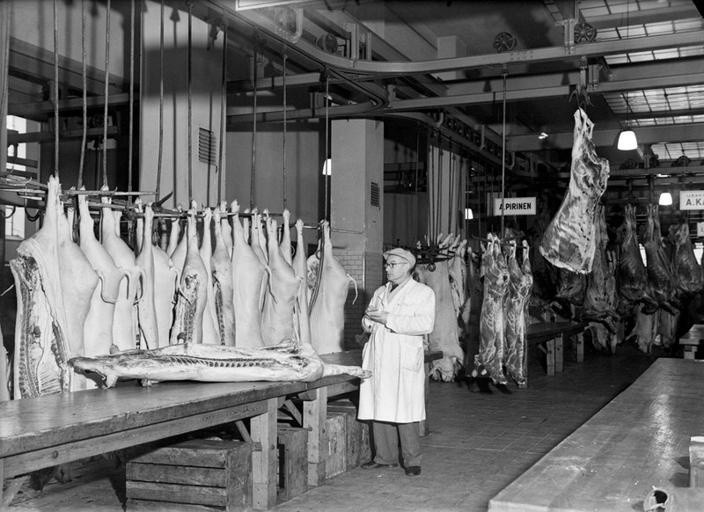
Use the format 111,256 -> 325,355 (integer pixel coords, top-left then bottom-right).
406,466 -> 421,475
361,461 -> 398,469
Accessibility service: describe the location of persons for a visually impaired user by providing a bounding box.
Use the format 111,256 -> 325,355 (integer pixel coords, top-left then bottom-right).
358,249 -> 435,476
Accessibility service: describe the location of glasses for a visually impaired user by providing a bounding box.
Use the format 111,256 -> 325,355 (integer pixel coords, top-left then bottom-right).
384,263 -> 407,267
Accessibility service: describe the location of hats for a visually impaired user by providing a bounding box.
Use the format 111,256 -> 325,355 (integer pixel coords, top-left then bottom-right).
383,248 -> 416,266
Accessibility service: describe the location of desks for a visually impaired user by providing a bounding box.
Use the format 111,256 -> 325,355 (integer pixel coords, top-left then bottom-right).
527,321 -> 589,375
2,346 -> 442,510
678,324 -> 704,360
487,354 -> 703,512
